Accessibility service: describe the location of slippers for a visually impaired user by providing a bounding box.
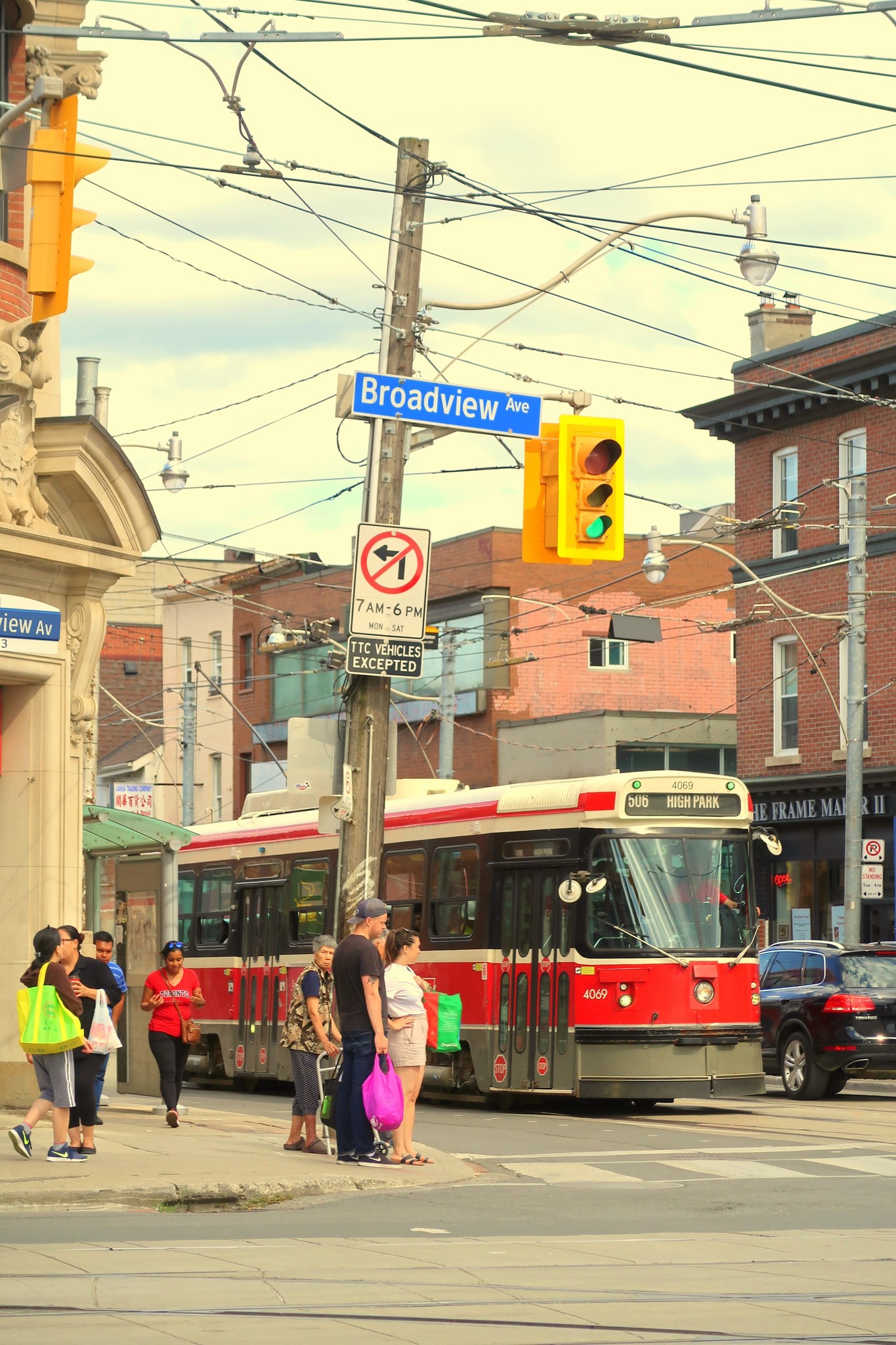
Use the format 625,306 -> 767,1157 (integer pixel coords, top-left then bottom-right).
415,1152 -> 434,1164
302,1138 -> 335,1155
391,1154 -> 425,1166
283,1136 -> 306,1150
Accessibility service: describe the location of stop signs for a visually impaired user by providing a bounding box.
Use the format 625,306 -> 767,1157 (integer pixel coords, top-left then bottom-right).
494,1054 -> 507,1082
501,957 -> 510,972
263,963 -> 269,975
241,963 -> 246,975
235,1045 -> 244,1069
537,1057 -> 548,1076
541,958 -> 550,971
260,1048 -> 266,1064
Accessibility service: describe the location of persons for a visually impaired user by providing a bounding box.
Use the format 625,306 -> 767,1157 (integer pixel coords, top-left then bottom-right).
94,931 -> 128,1124
331,897 -> 403,1170
141,940 -> 205,1128
369,927 -> 395,1149
8,924 -> 89,1162
667,853 -> 760,916
284,934 -> 342,1154
414,912 -> 422,932
59,926 -> 122,1155
382,926 -> 434,1166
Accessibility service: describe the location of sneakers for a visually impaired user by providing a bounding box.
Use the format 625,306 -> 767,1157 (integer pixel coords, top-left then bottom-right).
358,1150 -> 402,1169
47,1143 -> 88,1162
337,1152 -> 358,1166
8,1125 -> 33,1159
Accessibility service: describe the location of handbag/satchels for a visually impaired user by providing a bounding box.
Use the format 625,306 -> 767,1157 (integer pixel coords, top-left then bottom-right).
181,1019 -> 200,1045
422,986 -> 463,1052
320,1053 -> 345,1131
362,1052 -> 404,1131
17,961 -> 84,1056
88,988 -> 123,1056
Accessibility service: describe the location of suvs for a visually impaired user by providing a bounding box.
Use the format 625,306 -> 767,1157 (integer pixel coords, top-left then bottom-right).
759,939 -> 896,1099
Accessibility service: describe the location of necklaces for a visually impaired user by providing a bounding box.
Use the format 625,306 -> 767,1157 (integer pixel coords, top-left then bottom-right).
169,976 -> 175,986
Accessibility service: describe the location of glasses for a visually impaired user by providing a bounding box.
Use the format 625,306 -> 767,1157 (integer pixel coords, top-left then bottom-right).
401,926 -> 409,937
165,942 -> 184,948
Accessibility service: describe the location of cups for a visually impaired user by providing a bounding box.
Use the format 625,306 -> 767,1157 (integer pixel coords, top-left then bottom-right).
69,975 -> 80,996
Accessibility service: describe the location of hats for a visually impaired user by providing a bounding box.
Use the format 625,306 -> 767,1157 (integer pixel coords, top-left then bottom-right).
347,898 -> 388,925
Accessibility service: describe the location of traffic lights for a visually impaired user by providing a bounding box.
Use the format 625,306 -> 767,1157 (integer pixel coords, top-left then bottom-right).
557,414 -> 624,561
25,93 -> 110,323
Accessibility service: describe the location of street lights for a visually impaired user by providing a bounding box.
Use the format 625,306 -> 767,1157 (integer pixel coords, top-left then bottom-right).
332,196 -> 780,941
638,525 -> 862,950
260,616 -> 459,789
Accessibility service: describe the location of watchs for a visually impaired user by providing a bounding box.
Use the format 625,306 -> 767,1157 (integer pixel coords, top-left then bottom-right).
198,1000 -> 206,1008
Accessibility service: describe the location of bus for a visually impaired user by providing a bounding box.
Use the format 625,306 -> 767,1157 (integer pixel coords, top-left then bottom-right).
172,771 -> 782,1109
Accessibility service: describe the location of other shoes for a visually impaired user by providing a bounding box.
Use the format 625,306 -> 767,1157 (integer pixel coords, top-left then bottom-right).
95,1116 -> 103,1124
166,1109 -> 179,1128
69,1143 -> 96,1155
